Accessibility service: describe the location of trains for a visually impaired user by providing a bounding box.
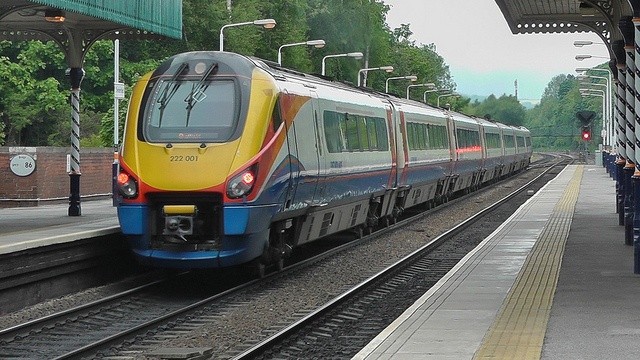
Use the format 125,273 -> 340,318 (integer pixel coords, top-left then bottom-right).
118,51 -> 532,278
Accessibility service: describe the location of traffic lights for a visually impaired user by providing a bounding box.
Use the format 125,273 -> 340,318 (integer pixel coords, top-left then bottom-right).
578,112 -> 595,140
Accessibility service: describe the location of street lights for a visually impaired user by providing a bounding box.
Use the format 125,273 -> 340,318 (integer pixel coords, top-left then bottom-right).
278,40 -> 326,67
579,88 -> 606,168
575,54 -> 611,62
437,94 -> 452,107
573,40 -> 606,47
575,67 -> 616,180
386,76 -> 417,93
581,93 -> 603,97
407,83 -> 435,100
578,75 -> 610,176
219,19 -> 276,51
322,52 -> 363,76
580,82 -> 609,173
358,66 -> 394,87
424,89 -> 438,103
